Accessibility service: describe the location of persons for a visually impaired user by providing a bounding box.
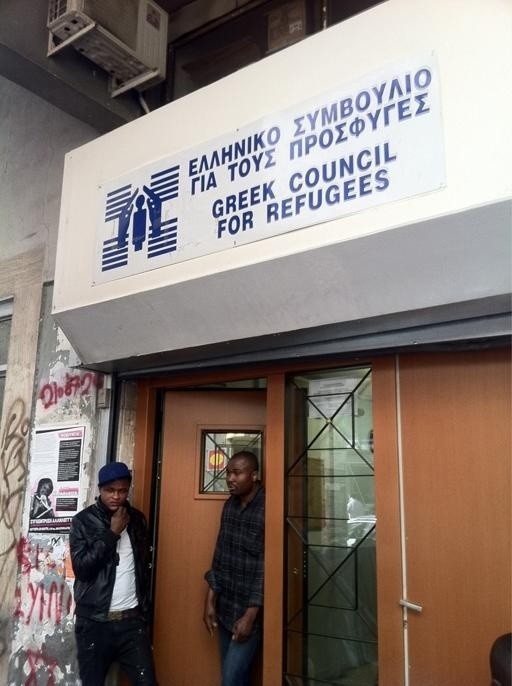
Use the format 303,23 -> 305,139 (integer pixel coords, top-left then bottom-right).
29,477 -> 55,518
68,461 -> 158,685
488,633 -> 511,685
202,449 -> 264,686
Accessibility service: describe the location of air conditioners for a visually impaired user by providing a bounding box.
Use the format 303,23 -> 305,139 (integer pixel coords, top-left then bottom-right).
48,0 -> 168,94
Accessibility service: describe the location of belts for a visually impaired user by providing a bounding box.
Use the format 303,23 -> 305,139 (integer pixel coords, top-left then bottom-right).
107,607 -> 140,621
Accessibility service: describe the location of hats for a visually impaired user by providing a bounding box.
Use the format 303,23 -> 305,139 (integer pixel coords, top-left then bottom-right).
97,462 -> 132,487
